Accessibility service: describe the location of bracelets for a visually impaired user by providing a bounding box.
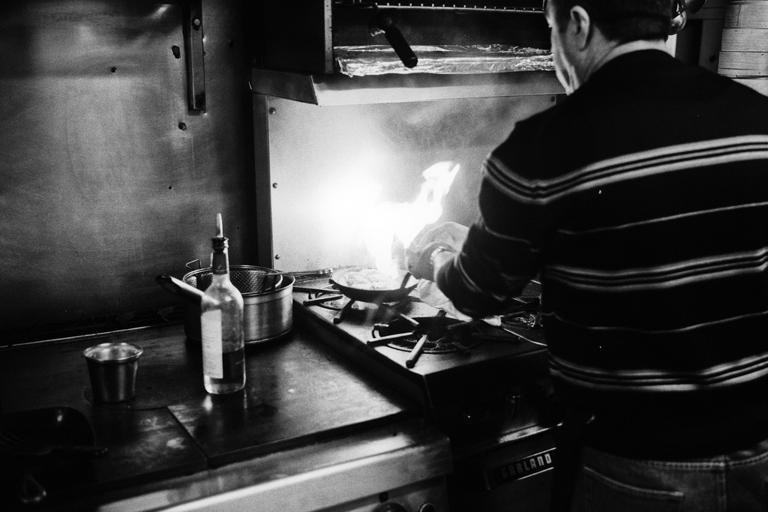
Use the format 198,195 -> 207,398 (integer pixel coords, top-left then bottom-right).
429,246 -> 453,282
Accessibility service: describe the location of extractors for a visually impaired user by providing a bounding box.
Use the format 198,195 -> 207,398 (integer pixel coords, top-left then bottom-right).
249,0 -> 569,108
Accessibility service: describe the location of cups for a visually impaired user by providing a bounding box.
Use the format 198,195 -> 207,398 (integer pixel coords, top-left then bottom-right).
82,340 -> 144,405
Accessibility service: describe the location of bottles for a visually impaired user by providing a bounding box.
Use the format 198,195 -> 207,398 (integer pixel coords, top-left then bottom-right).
199,237 -> 248,397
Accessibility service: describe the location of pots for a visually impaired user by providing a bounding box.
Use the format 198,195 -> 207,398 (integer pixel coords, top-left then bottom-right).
156,264 -> 297,345
328,264 -> 422,304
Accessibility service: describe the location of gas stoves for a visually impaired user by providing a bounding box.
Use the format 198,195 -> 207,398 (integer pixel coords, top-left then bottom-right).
282,268 -> 549,376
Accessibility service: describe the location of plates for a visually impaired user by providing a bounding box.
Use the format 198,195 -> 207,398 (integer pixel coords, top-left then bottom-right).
0,406 -> 97,491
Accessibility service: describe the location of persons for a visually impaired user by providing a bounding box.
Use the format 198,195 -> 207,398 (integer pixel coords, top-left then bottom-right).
409,0 -> 768,510
